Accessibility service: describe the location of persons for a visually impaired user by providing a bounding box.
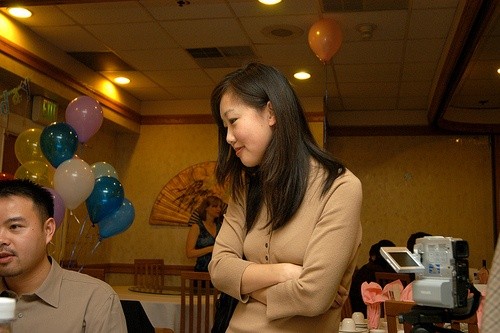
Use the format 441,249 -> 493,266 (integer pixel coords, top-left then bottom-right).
0,177 -> 127,333
348,240 -> 408,319
207,62 -> 363,332
185,197 -> 224,292
407,233 -> 432,283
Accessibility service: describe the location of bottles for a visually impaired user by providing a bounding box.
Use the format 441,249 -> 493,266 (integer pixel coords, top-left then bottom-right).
0,297 -> 16,333
479,259 -> 488,284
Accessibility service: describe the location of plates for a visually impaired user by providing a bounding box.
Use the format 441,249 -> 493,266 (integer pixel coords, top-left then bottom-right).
338,319 -> 370,333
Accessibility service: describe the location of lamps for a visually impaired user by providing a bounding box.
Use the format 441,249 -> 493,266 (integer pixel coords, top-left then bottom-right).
360,24 -> 375,39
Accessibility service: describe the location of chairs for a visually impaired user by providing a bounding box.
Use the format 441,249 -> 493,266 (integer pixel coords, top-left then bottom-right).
135,259 -> 165,289
374,271 -> 410,317
72,268 -> 105,281
180,270 -> 221,333
383,300 -> 481,333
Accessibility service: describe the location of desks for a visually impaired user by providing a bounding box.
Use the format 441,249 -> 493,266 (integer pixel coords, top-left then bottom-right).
110,284 -> 222,333
340,317 -> 481,332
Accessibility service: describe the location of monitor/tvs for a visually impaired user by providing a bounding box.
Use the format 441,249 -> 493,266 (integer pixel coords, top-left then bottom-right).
379,246 -> 425,274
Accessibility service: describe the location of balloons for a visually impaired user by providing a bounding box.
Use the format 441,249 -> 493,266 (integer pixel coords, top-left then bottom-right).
309,19 -> 344,65
0,122 -> 96,233
66,97 -> 104,145
86,160 -> 135,240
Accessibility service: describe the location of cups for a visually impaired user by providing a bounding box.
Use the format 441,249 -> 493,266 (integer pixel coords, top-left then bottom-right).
341,318 -> 356,331
352,312 -> 364,323
370,330 -> 385,333
